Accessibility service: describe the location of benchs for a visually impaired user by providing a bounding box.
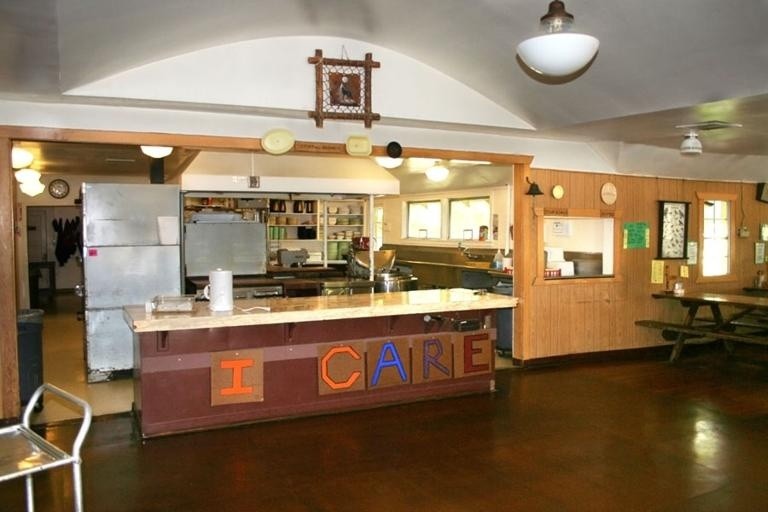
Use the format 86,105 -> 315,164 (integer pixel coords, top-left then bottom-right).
632,311 -> 768,366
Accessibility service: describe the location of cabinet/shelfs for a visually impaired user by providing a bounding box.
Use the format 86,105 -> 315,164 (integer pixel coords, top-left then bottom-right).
267,197 -> 369,269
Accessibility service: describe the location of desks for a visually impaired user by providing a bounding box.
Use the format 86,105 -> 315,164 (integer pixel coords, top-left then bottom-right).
651,284 -> 768,362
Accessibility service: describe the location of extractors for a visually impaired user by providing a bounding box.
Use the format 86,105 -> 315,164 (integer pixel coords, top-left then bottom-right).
180,151 -> 401,199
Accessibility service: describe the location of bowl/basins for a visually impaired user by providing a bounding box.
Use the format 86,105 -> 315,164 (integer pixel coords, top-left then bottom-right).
269,214 -> 298,226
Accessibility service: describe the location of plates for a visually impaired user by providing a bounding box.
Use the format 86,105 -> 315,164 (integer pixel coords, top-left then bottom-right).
327,204 -> 363,259
269,227 -> 288,241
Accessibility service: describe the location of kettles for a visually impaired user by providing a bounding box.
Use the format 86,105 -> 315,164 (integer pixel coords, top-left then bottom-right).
201,266 -> 234,312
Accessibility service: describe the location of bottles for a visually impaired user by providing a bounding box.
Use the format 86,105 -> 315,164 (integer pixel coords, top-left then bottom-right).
270,199 -> 315,213
200,196 -> 235,209
492,249 -> 513,274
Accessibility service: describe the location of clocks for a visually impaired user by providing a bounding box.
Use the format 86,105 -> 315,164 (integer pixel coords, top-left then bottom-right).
50,179 -> 70,199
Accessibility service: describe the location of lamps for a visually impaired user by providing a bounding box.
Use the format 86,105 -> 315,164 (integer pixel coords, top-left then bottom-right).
515,2 -> 602,79
526,177 -> 544,198
680,129 -> 705,156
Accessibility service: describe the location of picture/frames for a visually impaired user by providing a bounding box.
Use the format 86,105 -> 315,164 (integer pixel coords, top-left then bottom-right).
657,199 -> 692,262
